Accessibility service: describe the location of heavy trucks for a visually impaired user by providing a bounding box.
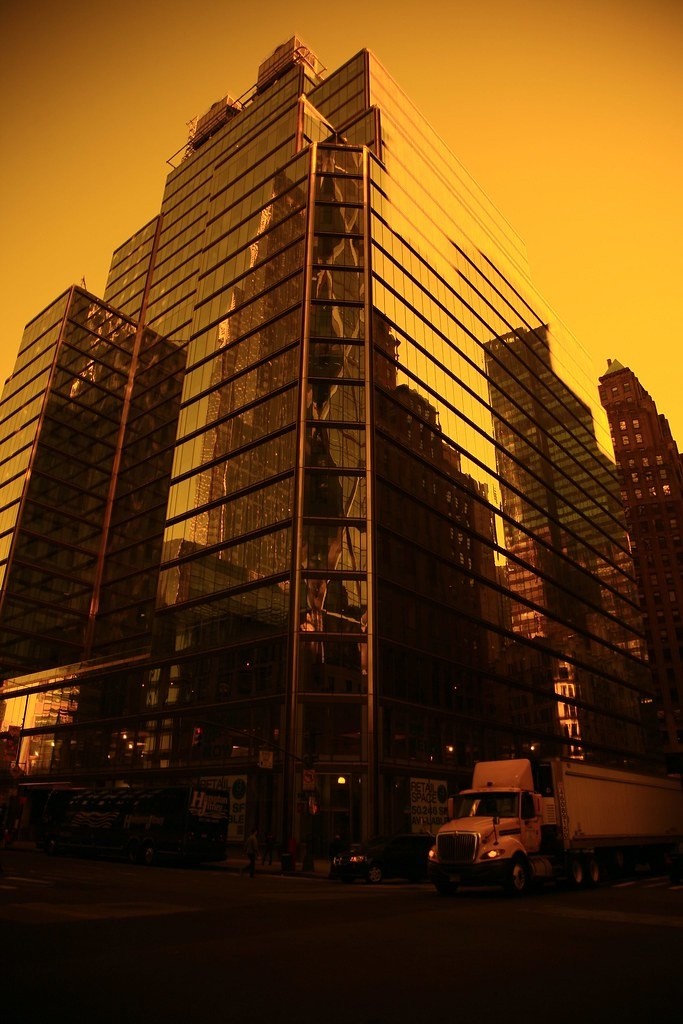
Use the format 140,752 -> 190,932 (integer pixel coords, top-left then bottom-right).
426,753 -> 683,899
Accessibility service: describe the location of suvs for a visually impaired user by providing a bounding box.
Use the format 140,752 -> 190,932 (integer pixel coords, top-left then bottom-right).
330,833 -> 438,885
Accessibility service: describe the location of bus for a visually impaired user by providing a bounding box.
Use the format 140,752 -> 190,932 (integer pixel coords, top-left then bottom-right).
40,785 -> 231,867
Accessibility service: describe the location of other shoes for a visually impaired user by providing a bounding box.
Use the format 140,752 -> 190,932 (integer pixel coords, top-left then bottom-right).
250,875 -> 256,878
240,867 -> 245,875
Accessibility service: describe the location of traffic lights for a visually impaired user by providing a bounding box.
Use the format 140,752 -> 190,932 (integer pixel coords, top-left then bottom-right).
193,726 -> 201,747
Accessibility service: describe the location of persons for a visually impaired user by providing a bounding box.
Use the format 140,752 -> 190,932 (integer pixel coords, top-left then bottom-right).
329,835 -> 344,880
303,834 -> 315,871
279,835 -> 296,875
240,829 -> 258,878
261,833 -> 274,865
